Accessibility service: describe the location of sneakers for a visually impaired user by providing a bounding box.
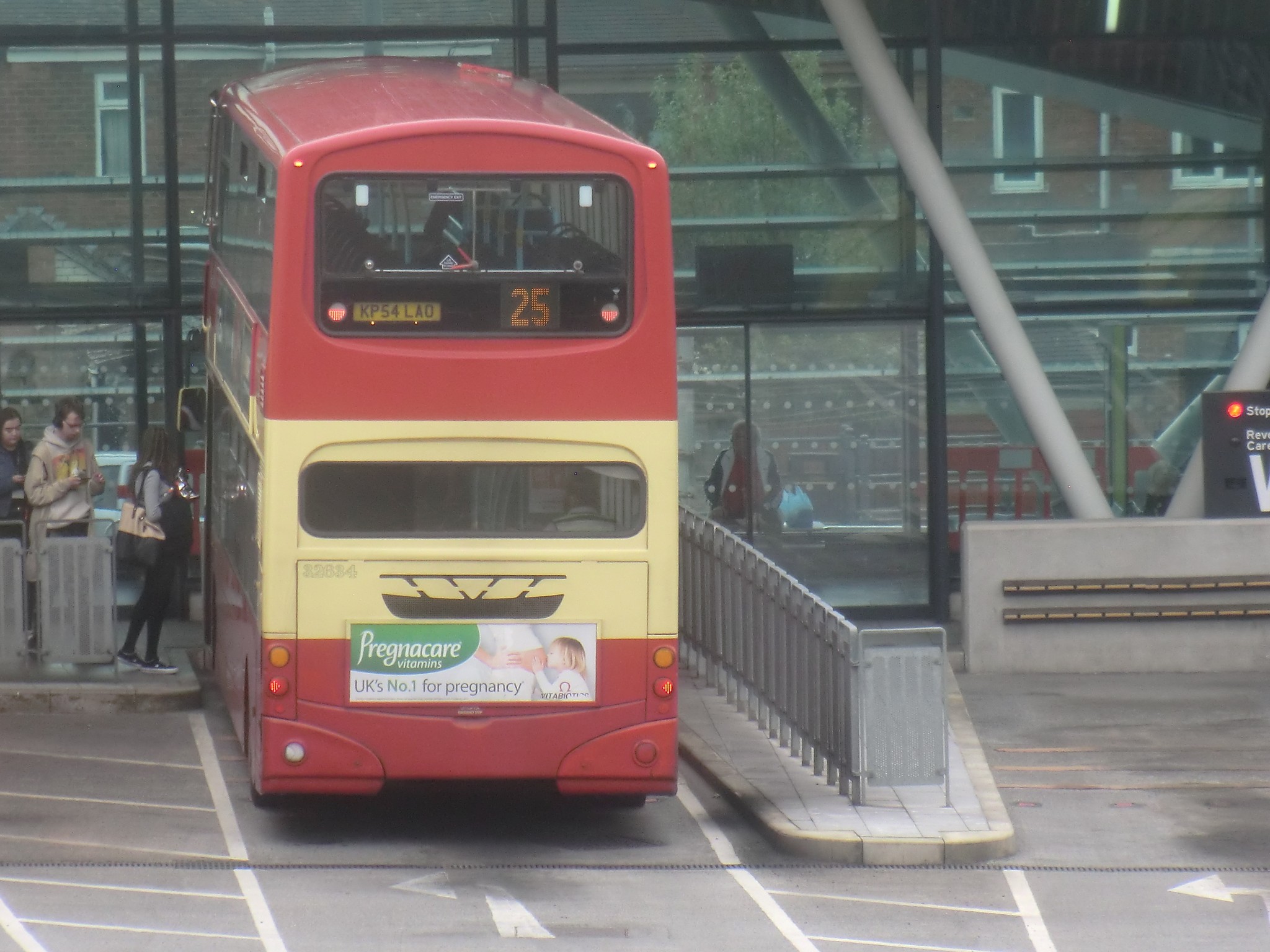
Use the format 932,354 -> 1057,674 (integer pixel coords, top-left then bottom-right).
141,659 -> 179,674
117,650 -> 146,668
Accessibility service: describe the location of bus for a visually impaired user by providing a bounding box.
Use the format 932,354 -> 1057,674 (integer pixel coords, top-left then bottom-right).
200,54 -> 679,814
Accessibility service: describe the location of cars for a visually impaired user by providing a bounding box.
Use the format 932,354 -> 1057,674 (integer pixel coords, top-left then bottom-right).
85,452 -> 138,534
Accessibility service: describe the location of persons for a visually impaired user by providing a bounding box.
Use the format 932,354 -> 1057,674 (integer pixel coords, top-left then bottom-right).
703,420 -> 785,533
117,425 -> 184,674
473,623 -> 591,701
25,396 -> 105,583
0,406 -> 37,640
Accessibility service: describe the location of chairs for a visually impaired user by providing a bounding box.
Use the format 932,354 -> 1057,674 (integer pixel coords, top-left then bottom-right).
233,191 -> 398,328
428,187 -> 624,330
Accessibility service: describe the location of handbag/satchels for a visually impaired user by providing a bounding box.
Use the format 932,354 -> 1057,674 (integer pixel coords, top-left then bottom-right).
777,486 -> 815,528
116,468 -> 166,569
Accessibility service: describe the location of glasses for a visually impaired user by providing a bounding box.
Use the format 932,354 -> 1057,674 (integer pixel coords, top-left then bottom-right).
63,420 -> 86,431
